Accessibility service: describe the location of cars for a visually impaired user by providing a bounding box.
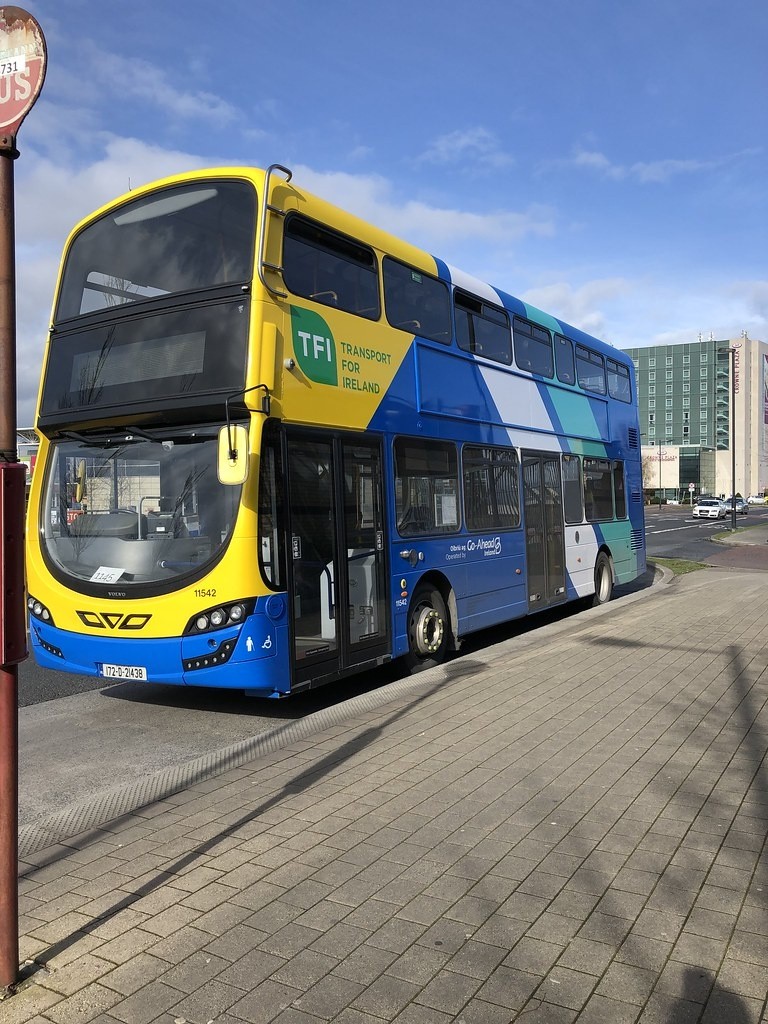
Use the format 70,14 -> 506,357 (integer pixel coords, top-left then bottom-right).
746,495 -> 768,505
723,497 -> 749,515
692,499 -> 727,520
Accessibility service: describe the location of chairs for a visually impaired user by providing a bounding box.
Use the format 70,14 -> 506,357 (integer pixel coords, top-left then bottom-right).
309,291 -> 627,401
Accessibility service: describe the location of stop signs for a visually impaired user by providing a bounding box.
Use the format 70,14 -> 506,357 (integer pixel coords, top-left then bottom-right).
0,2 -> 49,139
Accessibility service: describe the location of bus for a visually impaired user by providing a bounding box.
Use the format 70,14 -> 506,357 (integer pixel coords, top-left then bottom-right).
20,161 -> 650,703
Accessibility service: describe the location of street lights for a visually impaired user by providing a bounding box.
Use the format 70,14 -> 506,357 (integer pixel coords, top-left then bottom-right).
717,347 -> 737,533
657,438 -> 675,511
